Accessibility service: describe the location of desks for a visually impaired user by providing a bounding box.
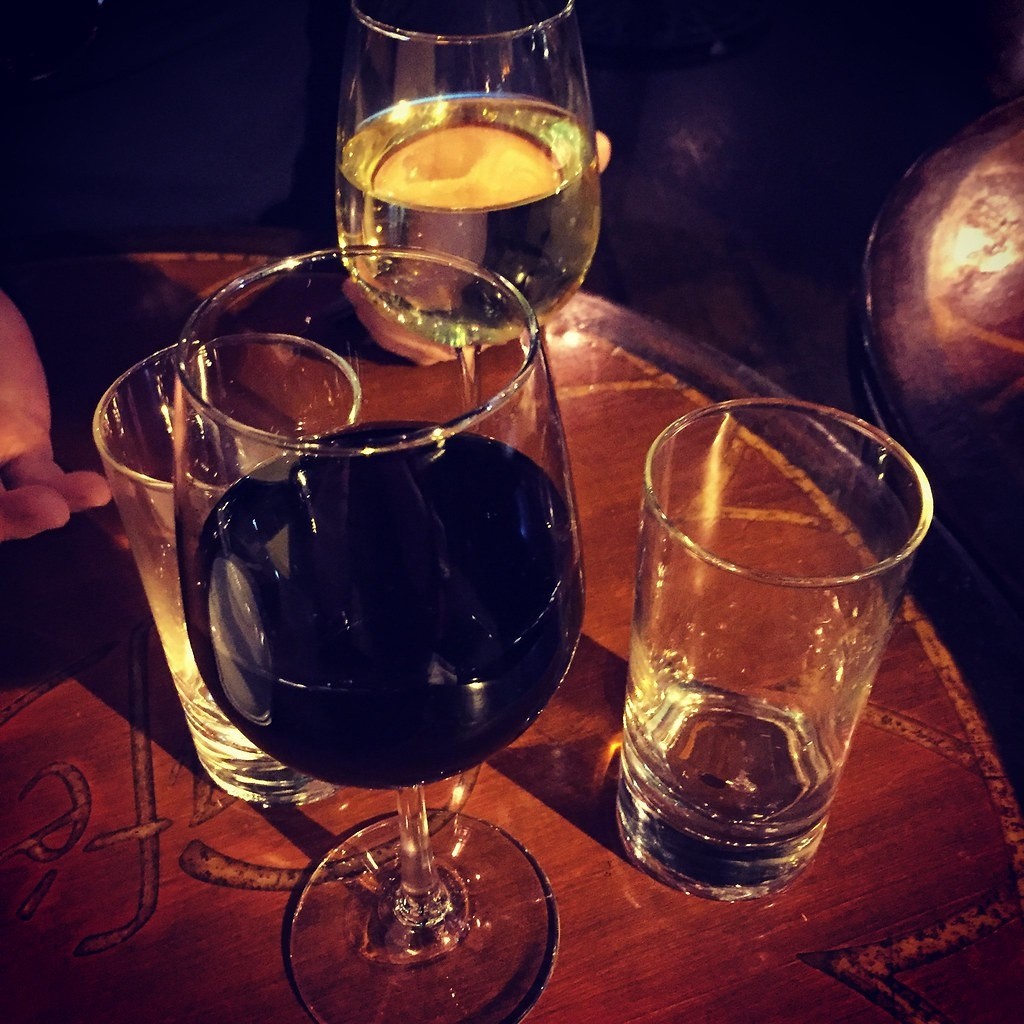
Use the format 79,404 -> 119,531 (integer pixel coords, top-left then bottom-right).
0,253 -> 1024,1024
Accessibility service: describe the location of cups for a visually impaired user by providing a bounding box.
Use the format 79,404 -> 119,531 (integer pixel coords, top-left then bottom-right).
615,396 -> 935,901
91,334 -> 367,806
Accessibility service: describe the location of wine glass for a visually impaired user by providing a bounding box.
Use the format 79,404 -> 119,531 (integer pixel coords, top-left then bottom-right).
334,1 -> 597,433
172,246 -> 587,1023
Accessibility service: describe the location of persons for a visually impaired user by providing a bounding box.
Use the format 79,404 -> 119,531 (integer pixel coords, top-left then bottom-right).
0,0 -> 773,544
